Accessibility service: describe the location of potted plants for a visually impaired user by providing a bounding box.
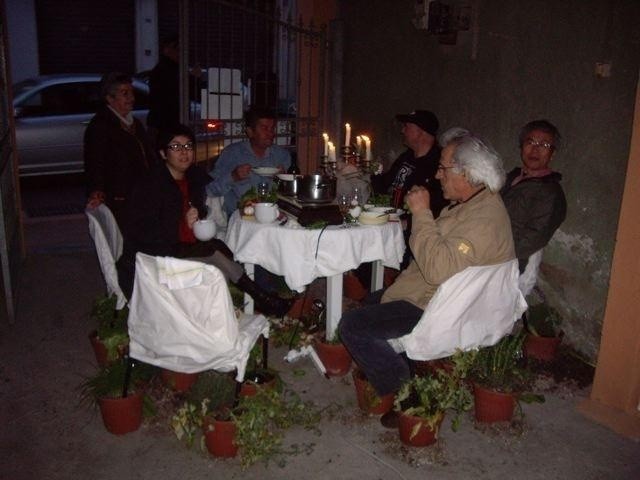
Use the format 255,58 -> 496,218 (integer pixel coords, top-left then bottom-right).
73,345 -> 318,472
399,300 -> 569,448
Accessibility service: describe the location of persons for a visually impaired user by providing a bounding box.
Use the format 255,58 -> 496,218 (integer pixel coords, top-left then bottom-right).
336,124 -> 521,430
147,37 -> 207,127
83,71 -> 163,212
207,105 -> 293,231
347,109 -> 451,293
121,122 -> 296,318
499,118 -> 570,278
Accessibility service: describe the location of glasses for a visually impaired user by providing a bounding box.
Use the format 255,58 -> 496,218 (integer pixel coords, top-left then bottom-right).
438,163 -> 455,173
527,140 -> 552,153
168,142 -> 193,152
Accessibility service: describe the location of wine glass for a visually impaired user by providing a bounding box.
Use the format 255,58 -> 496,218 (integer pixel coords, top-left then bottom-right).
338,187 -> 361,228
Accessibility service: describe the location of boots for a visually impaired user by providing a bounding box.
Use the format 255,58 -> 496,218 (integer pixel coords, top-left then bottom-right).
235,273 -> 289,315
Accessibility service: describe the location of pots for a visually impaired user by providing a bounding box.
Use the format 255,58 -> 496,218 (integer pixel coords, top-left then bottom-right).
295,174 -> 338,203
276,172 -> 300,197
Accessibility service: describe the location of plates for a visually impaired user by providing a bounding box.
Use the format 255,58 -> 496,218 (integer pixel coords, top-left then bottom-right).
356,205 -> 403,225
251,167 -> 280,176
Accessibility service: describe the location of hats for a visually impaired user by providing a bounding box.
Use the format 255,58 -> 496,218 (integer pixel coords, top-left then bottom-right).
395,110 -> 439,135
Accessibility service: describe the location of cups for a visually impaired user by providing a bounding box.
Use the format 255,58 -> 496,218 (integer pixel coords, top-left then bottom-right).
254,202 -> 279,223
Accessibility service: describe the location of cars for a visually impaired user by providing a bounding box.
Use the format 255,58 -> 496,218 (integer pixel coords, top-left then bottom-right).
6,74 -> 225,178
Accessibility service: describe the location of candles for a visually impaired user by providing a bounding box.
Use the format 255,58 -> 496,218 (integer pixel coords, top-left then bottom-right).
322,122 -> 372,162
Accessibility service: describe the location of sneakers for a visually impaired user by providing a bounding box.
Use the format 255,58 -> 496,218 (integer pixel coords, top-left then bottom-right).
381,408 -> 400,428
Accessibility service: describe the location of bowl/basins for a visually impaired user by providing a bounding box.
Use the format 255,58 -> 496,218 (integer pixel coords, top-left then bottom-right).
194,219 -> 216,242
